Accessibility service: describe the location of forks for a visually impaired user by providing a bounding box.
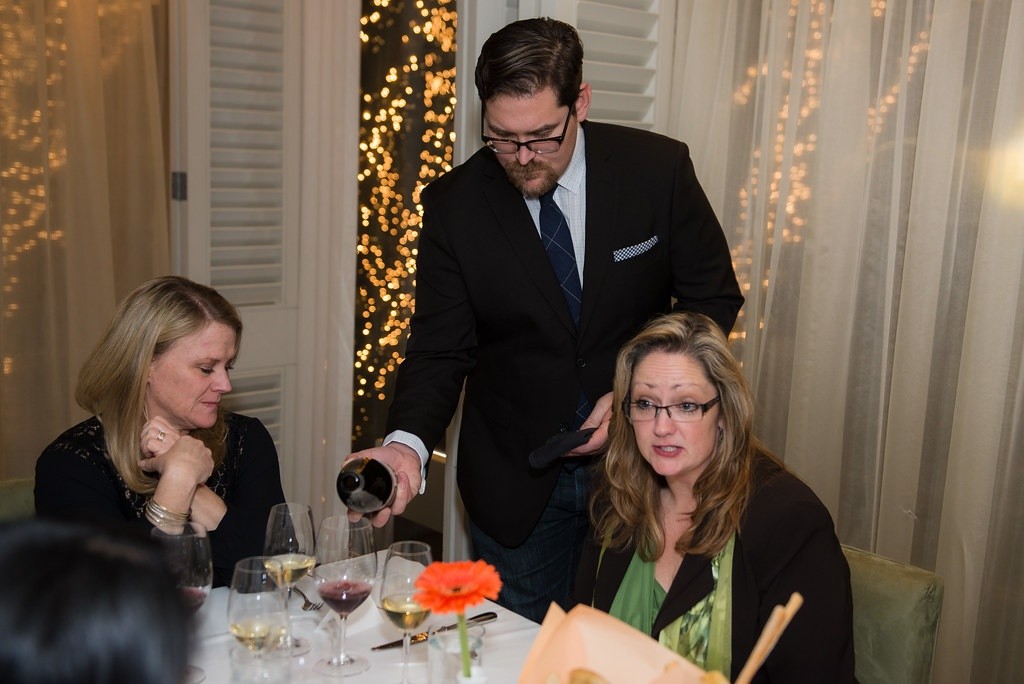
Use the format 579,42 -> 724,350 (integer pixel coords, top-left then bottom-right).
293,586 -> 325,610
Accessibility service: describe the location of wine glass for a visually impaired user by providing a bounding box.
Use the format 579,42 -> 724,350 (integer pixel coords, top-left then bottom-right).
148,518 -> 215,684
225,552 -> 288,659
263,501 -> 319,658
310,514 -> 378,678
425,615 -> 486,684
380,541 -> 433,684
227,609 -> 292,684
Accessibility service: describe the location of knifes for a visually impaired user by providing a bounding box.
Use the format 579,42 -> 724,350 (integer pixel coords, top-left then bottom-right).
370,612 -> 497,650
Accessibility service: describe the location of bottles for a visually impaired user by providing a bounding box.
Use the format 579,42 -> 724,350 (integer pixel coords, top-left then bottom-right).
335,458 -> 398,518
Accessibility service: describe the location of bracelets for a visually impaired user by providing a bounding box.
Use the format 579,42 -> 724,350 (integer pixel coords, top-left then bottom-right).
145,496 -> 193,528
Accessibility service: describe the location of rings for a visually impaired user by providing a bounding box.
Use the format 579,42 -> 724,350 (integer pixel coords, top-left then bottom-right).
156,430 -> 166,442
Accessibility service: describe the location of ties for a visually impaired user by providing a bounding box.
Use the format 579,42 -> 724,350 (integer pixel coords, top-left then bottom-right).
538,182 -> 583,331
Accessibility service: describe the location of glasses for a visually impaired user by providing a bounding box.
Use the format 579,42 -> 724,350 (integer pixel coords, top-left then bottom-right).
480,101 -> 576,154
622,392 -> 722,423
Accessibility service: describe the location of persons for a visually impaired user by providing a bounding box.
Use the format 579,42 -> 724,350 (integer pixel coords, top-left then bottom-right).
571,310 -> 862,684
33,274 -> 301,594
341,16 -> 748,626
1,515 -> 205,684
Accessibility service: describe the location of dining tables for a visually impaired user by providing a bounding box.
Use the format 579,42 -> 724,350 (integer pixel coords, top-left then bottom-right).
187,547 -> 543,684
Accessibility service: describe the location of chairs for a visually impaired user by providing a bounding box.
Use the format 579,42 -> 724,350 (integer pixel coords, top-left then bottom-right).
838,539 -> 947,684
